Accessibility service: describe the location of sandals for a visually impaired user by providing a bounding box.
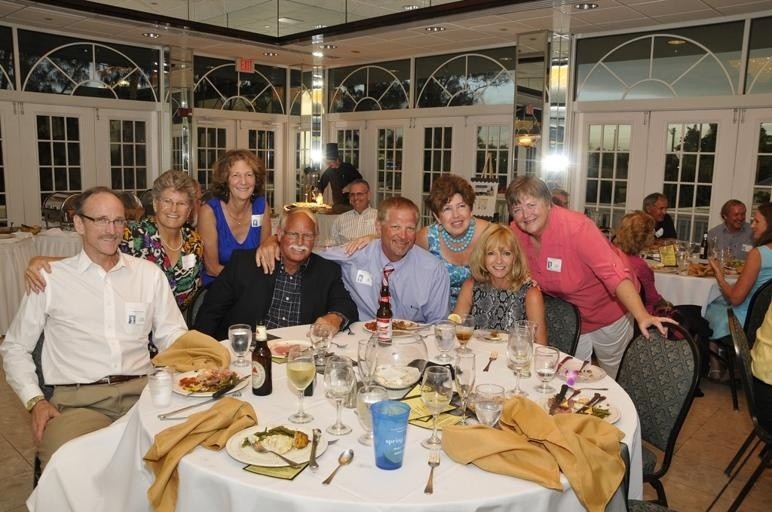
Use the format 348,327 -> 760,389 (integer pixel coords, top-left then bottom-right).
720,369 -> 742,385
703,370 -> 721,383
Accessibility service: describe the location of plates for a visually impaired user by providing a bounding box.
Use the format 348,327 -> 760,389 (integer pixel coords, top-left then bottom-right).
268,338 -> 316,360
362,318 -> 418,336
476,331 -> 511,341
227,424 -> 330,468
547,396 -> 620,426
170,368 -> 248,395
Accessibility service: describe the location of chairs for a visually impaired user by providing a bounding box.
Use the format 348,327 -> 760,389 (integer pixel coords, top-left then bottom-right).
541,295 -> 581,357
622,498 -> 678,512
704,306 -> 772,511
695,278 -> 772,411
614,320 -> 702,509
30,331 -> 54,490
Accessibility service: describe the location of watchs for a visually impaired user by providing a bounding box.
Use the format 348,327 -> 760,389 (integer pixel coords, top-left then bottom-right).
24,395 -> 45,413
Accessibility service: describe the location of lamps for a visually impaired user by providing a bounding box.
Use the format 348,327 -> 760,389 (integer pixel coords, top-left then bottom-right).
169,45 -> 194,117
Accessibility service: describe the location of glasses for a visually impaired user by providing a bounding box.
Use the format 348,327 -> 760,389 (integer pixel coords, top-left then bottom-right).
349,191 -> 369,197
77,213 -> 126,227
155,198 -> 191,209
283,230 -> 316,241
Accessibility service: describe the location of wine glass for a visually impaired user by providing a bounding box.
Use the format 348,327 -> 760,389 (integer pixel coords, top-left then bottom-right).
534,345 -> 560,396
511,319 -> 539,378
228,324 -> 252,366
286,346 -> 316,424
507,329 -> 531,399
323,355 -> 353,435
676,250 -> 686,276
421,366 -> 453,450
434,320 -> 456,362
454,354 -> 476,427
357,385 -> 388,445
453,314 -> 475,353
473,384 -> 505,428
358,339 -> 379,395
716,251 -> 727,269
309,323 -> 333,367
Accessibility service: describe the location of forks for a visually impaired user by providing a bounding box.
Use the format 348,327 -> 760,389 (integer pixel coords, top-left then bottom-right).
343,324 -> 356,338
424,448 -> 441,495
484,351 -> 499,372
251,441 -> 301,468
329,340 -> 348,349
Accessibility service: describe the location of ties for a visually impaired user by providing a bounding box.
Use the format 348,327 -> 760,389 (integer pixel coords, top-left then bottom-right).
378,265 -> 395,309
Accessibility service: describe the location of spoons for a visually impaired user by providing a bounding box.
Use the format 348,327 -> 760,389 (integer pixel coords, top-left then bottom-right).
323,448 -> 354,484
311,427 -> 322,467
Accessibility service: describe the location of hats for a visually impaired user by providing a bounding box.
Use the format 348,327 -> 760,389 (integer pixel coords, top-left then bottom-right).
326,143 -> 338,160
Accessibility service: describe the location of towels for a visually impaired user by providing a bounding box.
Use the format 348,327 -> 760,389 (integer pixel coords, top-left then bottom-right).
150,330 -> 231,372
142,396 -> 259,512
440,424 -> 565,493
493,394 -> 626,511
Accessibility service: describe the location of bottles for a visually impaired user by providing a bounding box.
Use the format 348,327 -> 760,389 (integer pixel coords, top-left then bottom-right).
376,288 -> 393,343
701,236 -> 708,259
250,322 -> 272,394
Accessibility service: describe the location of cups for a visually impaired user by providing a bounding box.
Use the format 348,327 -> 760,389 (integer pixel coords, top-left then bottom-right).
370,400 -> 411,470
147,367 -> 174,408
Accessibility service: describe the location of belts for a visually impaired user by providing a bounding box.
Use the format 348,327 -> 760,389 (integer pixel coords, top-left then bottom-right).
52,374 -> 148,387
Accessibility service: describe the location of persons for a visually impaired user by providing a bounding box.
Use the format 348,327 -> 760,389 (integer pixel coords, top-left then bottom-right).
750,297 -> 772,470
339,174 -> 494,316
194,148 -> 271,288
643,193 -> 677,240
192,206 -> 359,346
706,198 -> 755,262
453,223 -> 549,348
550,188 -> 570,209
505,174 -> 679,382
704,202 -> 772,388
25,169 -> 204,330
610,210 -> 673,326
327,180 -> 379,246
0,186 -> 188,476
256,196 -> 451,325
315,143 -> 362,212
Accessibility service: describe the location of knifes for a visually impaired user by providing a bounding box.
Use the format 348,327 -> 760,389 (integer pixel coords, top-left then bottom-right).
212,374 -> 251,398
548,383 -> 568,416
158,395 -> 225,418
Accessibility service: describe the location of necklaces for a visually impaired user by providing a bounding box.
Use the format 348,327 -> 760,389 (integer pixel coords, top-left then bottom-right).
439,218 -> 476,252
155,228 -> 185,253
225,203 -> 251,226
